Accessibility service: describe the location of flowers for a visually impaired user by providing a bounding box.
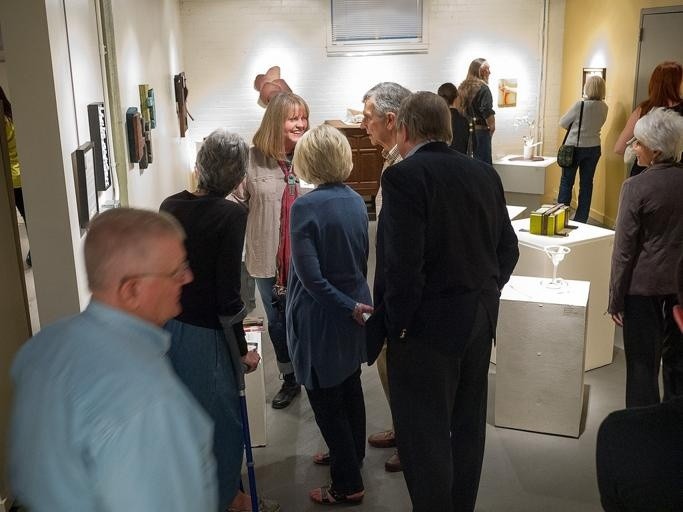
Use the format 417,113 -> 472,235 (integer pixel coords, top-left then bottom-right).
512,111 -> 543,147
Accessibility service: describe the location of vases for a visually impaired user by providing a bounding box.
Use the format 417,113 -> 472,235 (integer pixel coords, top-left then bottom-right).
522,146 -> 536,160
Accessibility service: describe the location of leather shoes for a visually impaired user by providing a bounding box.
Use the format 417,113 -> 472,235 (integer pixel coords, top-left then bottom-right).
368,430 -> 402,471
272,382 -> 300,409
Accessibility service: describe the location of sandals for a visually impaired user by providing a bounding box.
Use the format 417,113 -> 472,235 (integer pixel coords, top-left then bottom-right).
314,451 -> 332,464
311,486 -> 365,504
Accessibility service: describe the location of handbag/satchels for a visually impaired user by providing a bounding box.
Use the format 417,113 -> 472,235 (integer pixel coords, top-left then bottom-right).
557,145 -> 577,166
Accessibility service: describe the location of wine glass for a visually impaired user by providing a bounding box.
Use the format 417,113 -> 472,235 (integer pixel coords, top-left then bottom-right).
544,246 -> 571,289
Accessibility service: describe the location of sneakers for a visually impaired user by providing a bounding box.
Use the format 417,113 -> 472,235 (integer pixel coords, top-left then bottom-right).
228,494 -> 281,512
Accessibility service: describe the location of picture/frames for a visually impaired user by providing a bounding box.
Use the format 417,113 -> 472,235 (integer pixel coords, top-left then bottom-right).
581,67 -> 607,102
70,141 -> 101,229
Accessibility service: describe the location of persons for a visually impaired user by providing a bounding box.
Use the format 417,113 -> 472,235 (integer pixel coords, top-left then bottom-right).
596,61 -> 683,512
557,75 -> 608,223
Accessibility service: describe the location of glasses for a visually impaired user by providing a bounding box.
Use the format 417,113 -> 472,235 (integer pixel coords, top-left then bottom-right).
138,260 -> 189,283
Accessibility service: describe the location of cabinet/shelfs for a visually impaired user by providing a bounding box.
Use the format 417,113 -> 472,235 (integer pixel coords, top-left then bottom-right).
329,124 -> 381,210
